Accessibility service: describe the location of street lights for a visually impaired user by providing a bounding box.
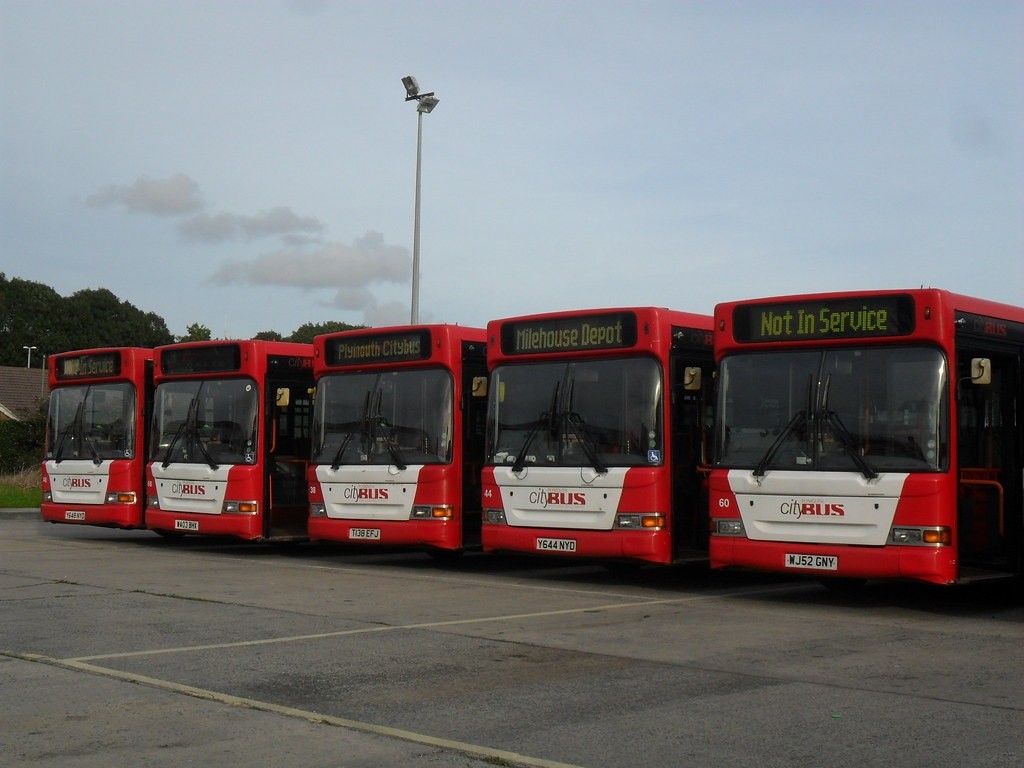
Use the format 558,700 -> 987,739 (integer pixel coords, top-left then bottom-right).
401,75 -> 440,325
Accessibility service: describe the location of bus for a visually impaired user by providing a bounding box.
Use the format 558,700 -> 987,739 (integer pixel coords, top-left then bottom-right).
40,347 -> 153,529
150,341 -> 315,545
483,304 -> 793,572
306,323 -> 509,560
706,288 -> 1024,606
